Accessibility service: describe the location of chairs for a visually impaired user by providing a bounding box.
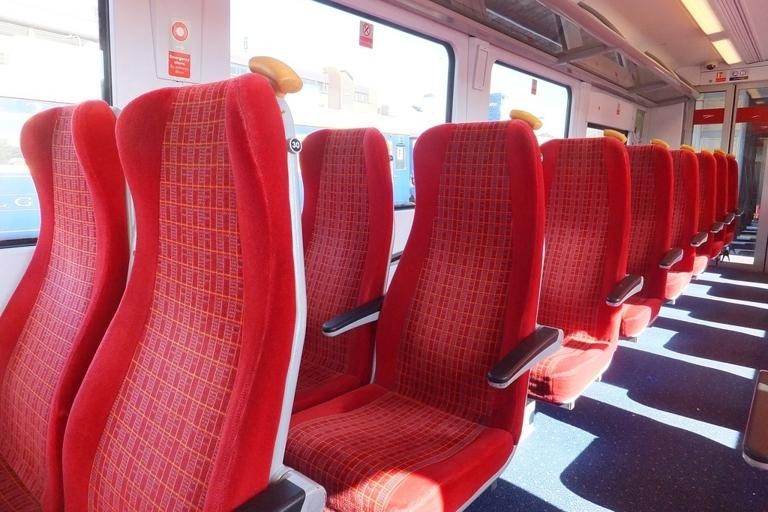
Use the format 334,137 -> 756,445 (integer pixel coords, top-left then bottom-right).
61,72 -> 331,511
293,127 -> 395,410
0,98 -> 128,511
289,118 -> 566,512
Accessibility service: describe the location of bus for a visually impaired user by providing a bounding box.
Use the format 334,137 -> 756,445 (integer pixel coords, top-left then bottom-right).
295,124 -> 422,209
